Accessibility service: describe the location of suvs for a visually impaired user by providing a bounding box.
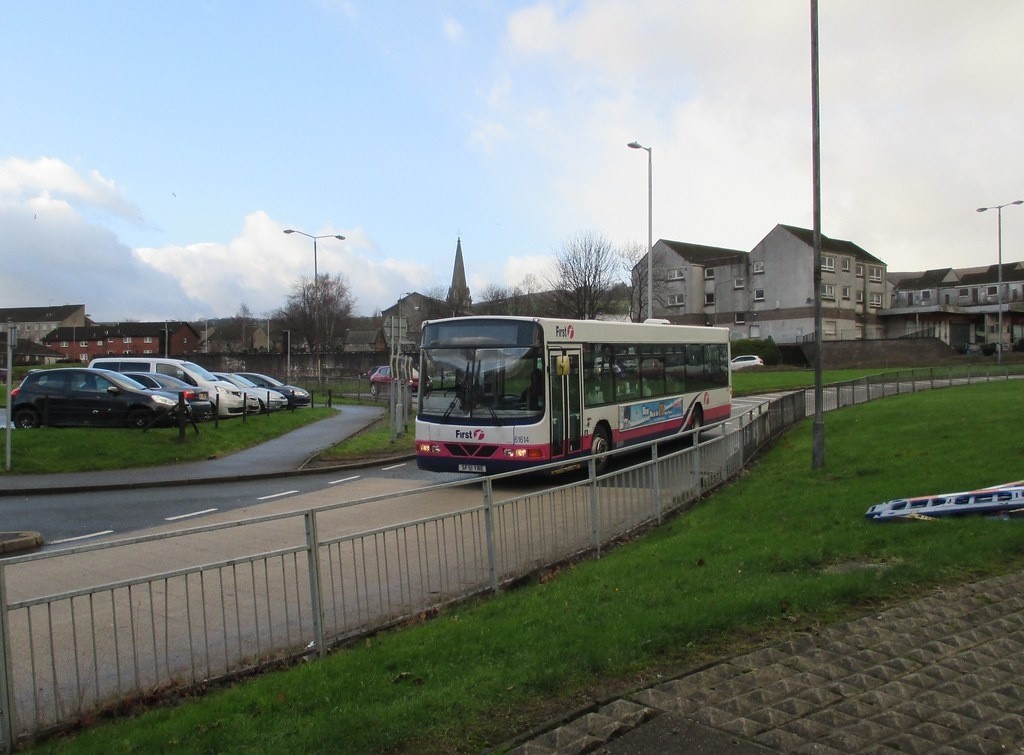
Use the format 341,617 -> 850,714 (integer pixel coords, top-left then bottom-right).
10,367 -> 192,430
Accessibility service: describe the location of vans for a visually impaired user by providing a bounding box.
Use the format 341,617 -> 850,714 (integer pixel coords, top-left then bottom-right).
87,357 -> 247,421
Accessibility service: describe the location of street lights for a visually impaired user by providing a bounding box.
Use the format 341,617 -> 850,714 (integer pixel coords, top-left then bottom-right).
398,292 -> 412,345
283,329 -> 291,385
284,229 -> 345,376
73,314 -> 91,362
975,200 -> 1024,362
164,319 -> 178,358
627,141 -> 653,319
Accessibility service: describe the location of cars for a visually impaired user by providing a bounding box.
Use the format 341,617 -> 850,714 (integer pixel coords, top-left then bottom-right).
76,371 -> 212,423
369,365 -> 434,396
720,355 -> 764,372
366,366 -> 383,379
210,372 -> 289,414
233,372 -> 312,410
212,373 -> 260,413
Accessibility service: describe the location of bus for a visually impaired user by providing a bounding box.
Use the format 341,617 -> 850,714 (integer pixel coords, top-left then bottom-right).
414,316 -> 734,480
